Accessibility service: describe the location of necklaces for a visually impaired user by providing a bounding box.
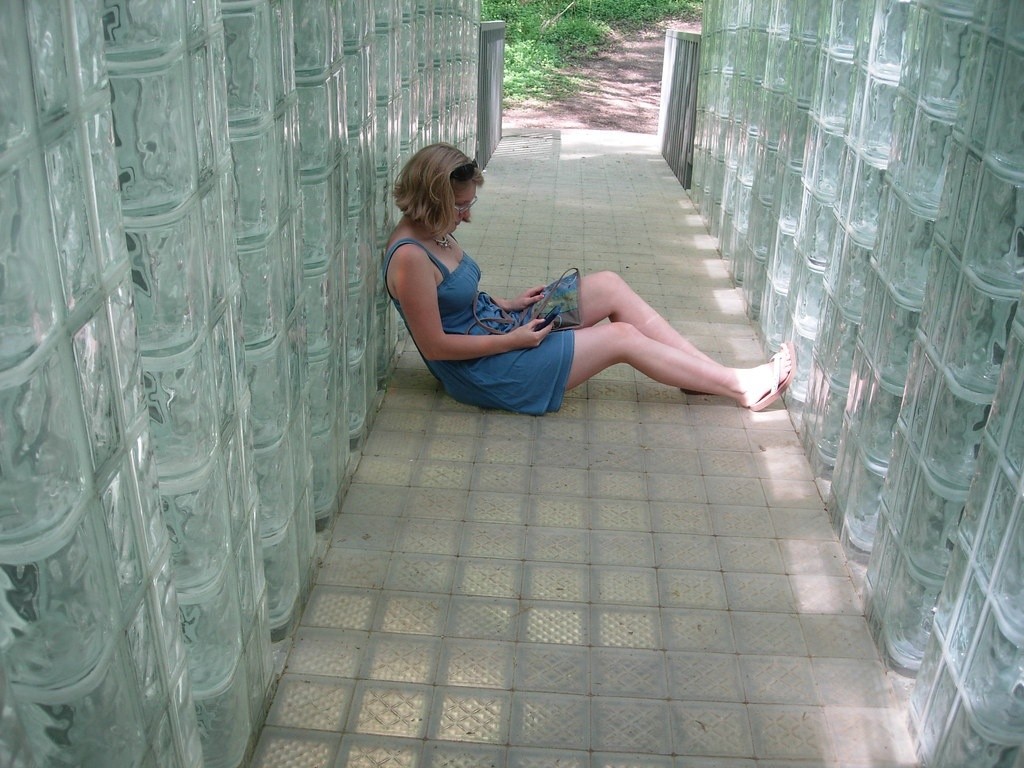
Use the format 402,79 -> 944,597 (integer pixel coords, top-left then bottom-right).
431,235 -> 453,249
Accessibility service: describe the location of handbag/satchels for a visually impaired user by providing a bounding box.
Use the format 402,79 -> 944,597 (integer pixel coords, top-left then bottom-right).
463,267 -> 584,335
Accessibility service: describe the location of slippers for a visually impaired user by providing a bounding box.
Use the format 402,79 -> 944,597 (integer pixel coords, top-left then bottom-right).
750,340 -> 797,412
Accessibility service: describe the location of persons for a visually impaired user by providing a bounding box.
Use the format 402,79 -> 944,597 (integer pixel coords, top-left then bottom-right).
382,144 -> 796,416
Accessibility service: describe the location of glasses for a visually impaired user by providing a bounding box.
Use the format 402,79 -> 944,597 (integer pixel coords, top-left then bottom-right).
454,195 -> 478,214
449,158 -> 479,183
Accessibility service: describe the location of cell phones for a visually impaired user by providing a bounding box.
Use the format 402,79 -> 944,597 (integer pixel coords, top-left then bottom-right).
534,306 -> 561,332
